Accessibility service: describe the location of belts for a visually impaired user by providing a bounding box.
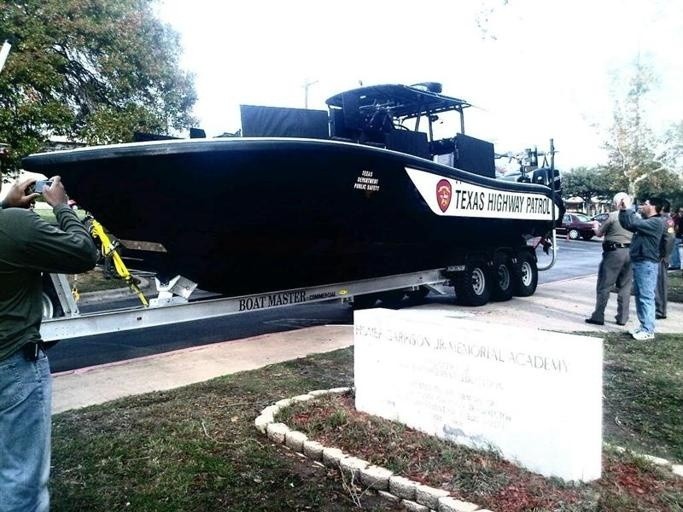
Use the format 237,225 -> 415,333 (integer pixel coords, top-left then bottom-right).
616,244 -> 630,248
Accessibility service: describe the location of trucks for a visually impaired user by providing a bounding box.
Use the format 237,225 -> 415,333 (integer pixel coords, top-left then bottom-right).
1,182 -> 538,356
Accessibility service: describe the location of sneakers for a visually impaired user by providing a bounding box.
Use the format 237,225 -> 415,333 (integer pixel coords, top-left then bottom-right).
627,329 -> 655,341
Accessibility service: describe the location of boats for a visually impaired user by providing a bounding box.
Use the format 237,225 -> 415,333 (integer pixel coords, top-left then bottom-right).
27,83 -> 567,295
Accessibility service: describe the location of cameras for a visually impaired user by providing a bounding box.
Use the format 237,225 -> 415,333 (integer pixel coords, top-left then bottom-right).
35,181 -> 51,193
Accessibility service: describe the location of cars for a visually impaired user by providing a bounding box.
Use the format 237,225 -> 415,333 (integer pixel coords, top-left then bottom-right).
555,213 -> 613,241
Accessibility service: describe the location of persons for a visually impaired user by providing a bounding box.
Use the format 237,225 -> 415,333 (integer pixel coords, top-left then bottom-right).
584,192 -> 635,326
618,197 -> 666,342
637,199 -> 683,320
0,175 -> 98,512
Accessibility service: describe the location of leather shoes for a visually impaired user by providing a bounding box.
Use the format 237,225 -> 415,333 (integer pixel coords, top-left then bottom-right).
616,321 -> 625,325
585,319 -> 604,325
656,315 -> 666,319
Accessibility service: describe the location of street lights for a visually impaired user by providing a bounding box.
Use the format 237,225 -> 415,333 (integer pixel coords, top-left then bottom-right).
305,79 -> 319,109
